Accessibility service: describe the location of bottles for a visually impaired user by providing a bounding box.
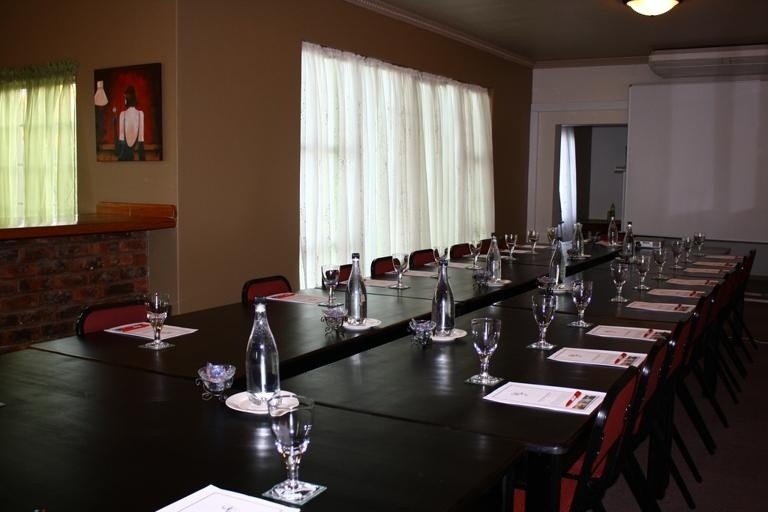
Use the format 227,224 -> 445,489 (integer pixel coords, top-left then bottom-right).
486,232 -> 501,282
549,237 -> 566,289
608,214 -> 618,246
432,260 -> 455,337
623,221 -> 634,254
245,296 -> 280,405
572,223 -> 584,256
346,253 -> 367,325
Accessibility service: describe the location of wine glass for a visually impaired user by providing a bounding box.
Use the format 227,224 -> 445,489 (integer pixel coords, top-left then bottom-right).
682,235 -> 694,264
389,249 -> 412,292
143,289 -> 173,351
318,265 -> 343,310
632,242 -> 653,290
607,259 -> 630,303
502,234 -> 516,261
653,241 -> 668,281
545,225 -> 557,252
569,280 -> 596,328
694,232 -> 706,257
671,240 -> 685,269
525,294 -> 556,352
467,239 -> 483,271
264,392 -> 318,501
464,317 -> 504,387
431,246 -> 451,280
525,228 -> 539,254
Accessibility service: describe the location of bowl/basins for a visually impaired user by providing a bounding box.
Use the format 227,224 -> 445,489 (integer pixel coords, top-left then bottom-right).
472,270 -> 491,288
197,364 -> 236,392
323,308 -> 348,329
537,276 -> 553,289
410,320 -> 437,339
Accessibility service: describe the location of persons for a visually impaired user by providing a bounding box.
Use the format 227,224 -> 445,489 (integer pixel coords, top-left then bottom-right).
113,85 -> 145,160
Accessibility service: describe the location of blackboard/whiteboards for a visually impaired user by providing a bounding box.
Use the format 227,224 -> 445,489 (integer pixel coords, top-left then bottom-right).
622,79 -> 768,244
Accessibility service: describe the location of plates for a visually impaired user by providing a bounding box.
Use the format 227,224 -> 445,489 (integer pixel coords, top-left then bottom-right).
343,318 -> 382,332
574,255 -> 591,260
487,280 -> 512,287
553,284 -> 571,294
225,390 -> 299,414
432,329 -> 467,342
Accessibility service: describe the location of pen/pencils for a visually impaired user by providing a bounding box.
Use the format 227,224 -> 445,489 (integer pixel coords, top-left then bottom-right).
674,304 -> 681,310
615,353 -> 626,364
566,391 -> 581,407
644,330 -> 652,337
